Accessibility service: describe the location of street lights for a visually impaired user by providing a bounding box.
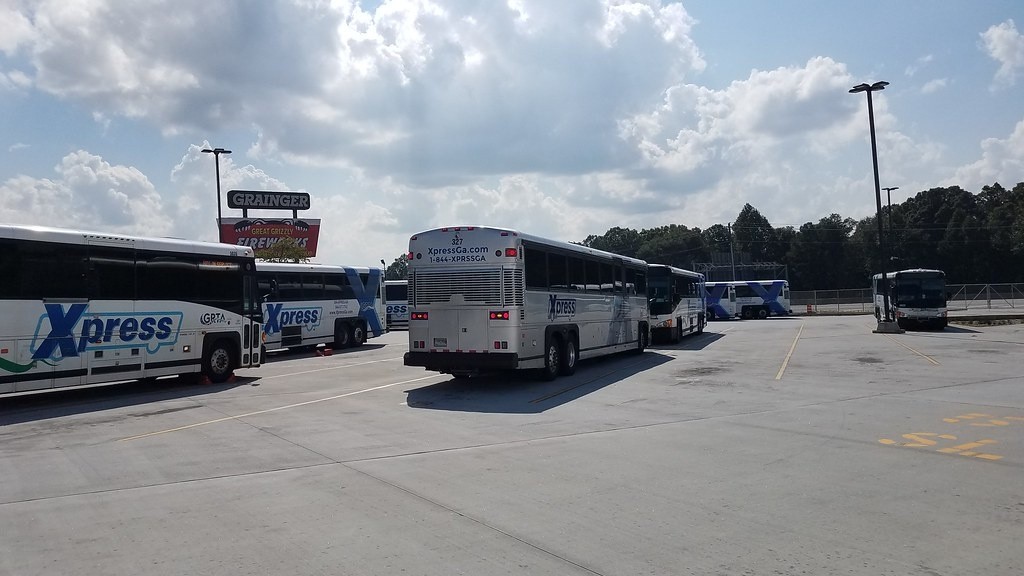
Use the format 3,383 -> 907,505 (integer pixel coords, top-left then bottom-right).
201,148 -> 232,243
882,187 -> 900,271
848,81 -> 905,333
381,260 -> 385,273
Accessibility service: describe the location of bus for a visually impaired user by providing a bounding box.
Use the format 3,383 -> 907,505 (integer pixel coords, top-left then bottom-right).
255,262 -> 390,351
403,226 -> 656,380
735,280 -> 790,320
384,280 -> 409,326
705,282 -> 736,321
0,227 -> 278,399
648,264 -> 708,344
551,282 -> 636,296
872,268 -> 948,330
